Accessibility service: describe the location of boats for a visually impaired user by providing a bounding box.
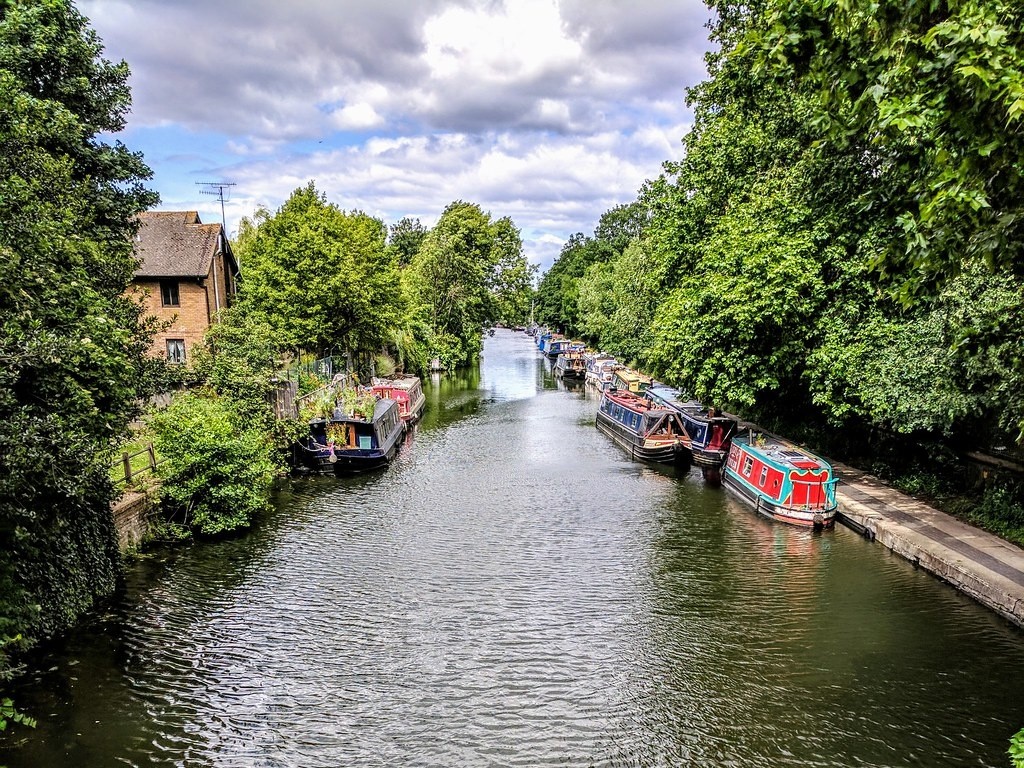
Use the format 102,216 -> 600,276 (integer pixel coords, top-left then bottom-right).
718,427 -> 839,530
301,393 -> 408,477
593,385 -> 694,478
364,374 -> 427,433
525,319 -> 653,399
643,387 -> 740,469
